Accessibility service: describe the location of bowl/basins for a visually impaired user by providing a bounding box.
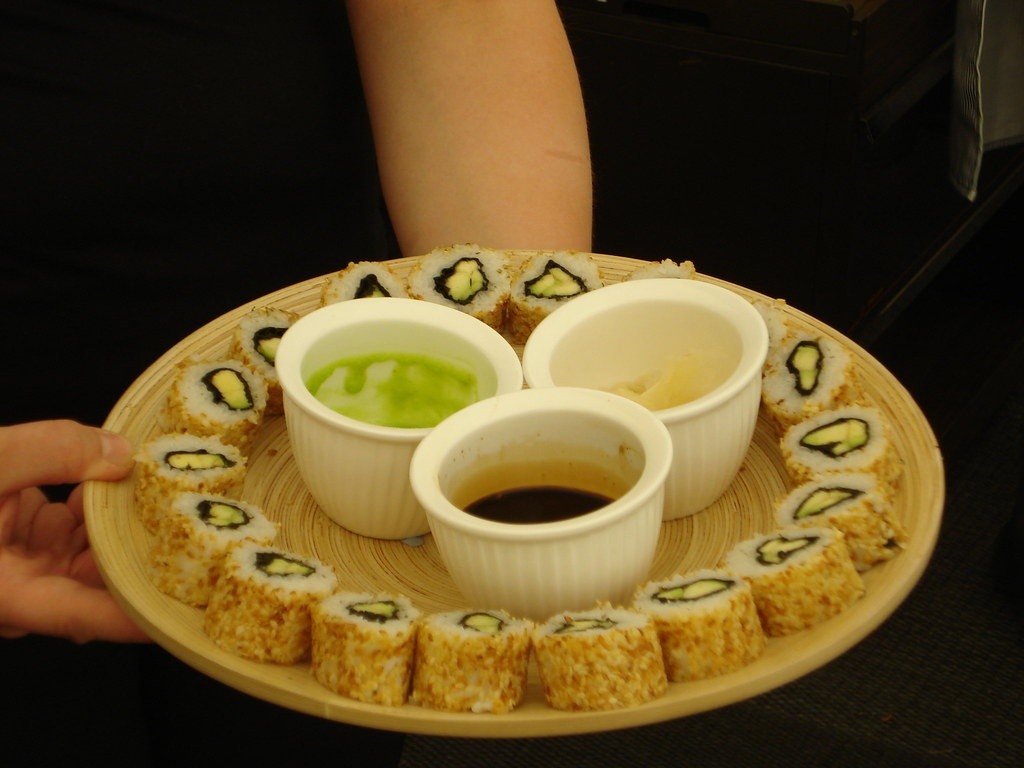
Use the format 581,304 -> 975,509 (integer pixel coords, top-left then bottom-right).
407,387 -> 673,622
521,277 -> 771,522
274,296 -> 524,540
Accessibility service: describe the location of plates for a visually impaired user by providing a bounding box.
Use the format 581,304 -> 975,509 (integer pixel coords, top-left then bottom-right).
82,247 -> 946,740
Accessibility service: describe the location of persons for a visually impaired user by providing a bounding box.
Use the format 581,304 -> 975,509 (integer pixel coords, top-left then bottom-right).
0,0 -> 594,645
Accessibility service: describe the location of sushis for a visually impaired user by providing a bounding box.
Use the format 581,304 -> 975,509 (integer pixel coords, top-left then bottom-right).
129,242 -> 904,713
